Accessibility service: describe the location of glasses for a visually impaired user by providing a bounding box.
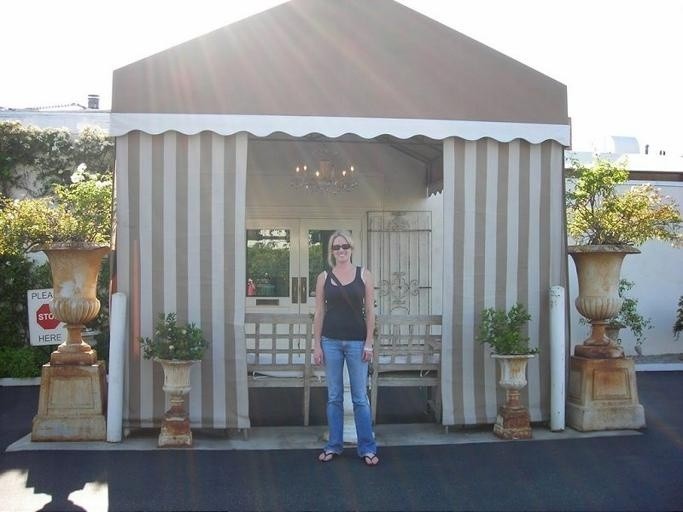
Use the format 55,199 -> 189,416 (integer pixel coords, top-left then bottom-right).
332,244 -> 350,250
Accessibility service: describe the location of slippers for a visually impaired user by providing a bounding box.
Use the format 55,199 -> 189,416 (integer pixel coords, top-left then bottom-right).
321,451 -> 333,462
364,456 -> 376,465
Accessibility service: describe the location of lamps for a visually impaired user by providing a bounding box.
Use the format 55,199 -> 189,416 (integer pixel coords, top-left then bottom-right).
288,152 -> 359,195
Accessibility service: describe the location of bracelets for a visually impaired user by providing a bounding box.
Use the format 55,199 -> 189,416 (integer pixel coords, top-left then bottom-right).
362,346 -> 374,353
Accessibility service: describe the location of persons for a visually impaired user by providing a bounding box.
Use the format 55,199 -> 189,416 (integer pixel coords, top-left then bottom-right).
246,278 -> 256,295
312,228 -> 380,470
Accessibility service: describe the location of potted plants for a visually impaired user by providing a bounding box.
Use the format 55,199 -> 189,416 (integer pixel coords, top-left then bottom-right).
565,154 -> 683,357
0,168 -> 113,365
136,310 -> 207,401
472,305 -> 542,391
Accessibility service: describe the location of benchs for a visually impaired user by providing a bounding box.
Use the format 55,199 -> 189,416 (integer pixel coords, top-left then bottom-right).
245,314 -> 440,426
